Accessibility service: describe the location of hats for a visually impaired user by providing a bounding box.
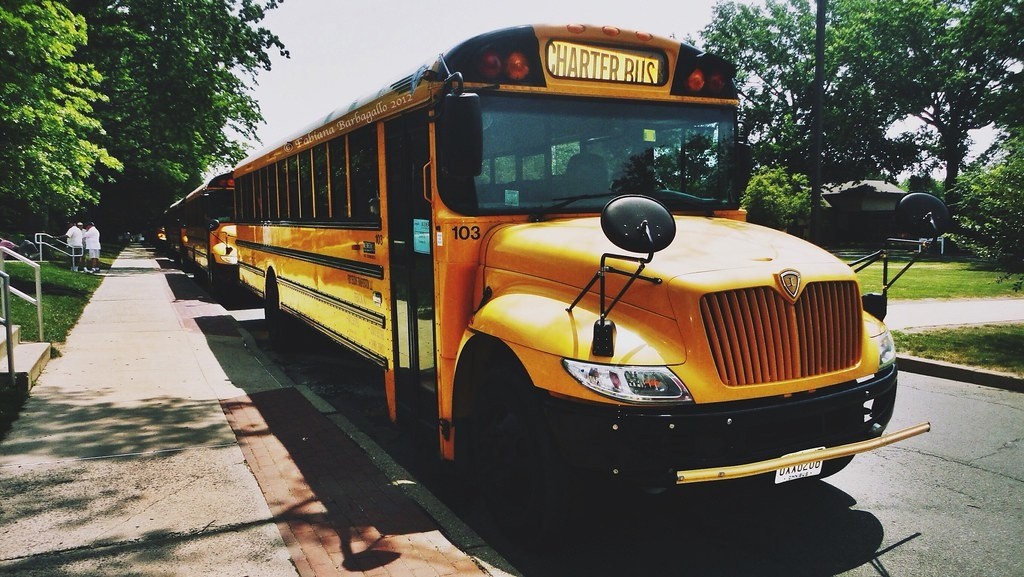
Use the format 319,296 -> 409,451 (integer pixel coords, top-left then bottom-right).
77,222 -> 83,225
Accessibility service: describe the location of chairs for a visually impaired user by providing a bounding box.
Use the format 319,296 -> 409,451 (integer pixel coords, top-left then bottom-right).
560,154 -> 612,204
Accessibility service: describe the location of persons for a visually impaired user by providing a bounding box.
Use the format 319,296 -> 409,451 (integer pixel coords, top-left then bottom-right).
610,144 -> 659,193
51,221 -> 101,273
0,238 -> 39,259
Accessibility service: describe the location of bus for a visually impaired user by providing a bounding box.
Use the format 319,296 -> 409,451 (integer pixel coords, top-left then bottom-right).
156,22 -> 949,543
